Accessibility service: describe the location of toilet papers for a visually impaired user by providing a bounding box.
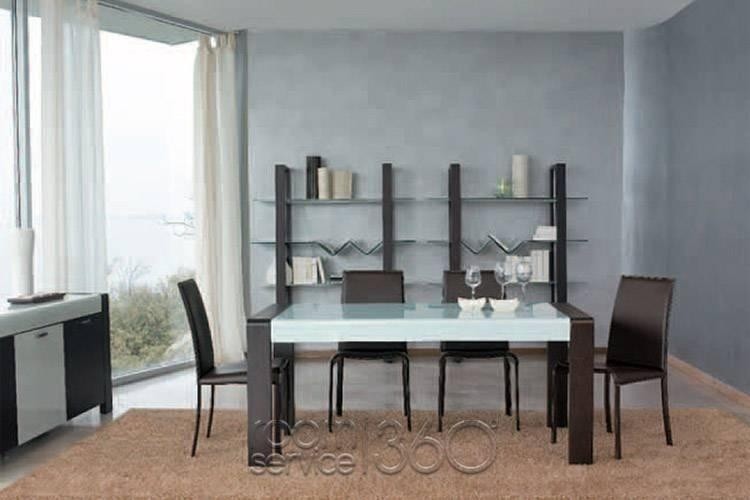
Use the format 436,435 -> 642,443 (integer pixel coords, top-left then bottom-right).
318,167 -> 331,200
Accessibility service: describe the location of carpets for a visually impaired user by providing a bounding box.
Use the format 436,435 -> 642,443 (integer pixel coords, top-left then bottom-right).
0,409 -> 749,500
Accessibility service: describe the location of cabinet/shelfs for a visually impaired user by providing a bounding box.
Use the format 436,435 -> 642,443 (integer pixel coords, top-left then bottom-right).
250,156 -> 417,364
426,163 -> 588,350
0,291 -> 113,457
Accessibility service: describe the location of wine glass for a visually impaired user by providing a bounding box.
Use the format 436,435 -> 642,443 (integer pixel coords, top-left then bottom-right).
465,262 -> 533,309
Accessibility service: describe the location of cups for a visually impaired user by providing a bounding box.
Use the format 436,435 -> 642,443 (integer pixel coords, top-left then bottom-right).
493,176 -> 511,197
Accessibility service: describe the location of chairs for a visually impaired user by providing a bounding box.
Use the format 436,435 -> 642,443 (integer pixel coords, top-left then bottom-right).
177,278 -> 290,457
551,274 -> 677,458
328,271 -> 413,433
437,270 -> 521,433
